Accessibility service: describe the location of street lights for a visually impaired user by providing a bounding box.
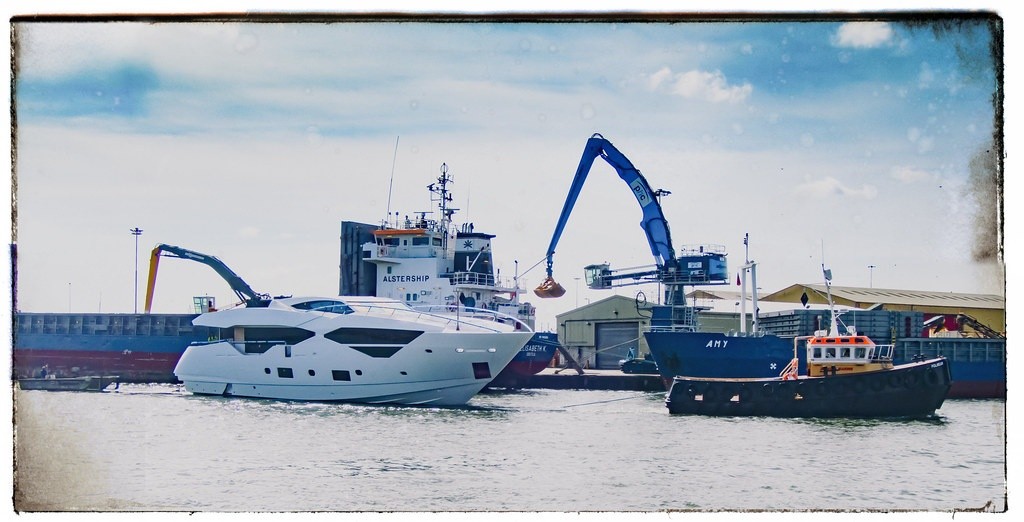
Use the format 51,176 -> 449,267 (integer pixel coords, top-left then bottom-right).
867,265 -> 876,288
129,227 -> 143,314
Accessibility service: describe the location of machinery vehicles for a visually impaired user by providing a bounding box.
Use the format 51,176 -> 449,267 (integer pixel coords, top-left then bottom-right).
144,243 -> 269,314
531,133 -> 731,375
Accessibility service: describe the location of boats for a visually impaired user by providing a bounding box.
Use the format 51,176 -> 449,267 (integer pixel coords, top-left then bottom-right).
16,374 -> 122,393
173,293 -> 534,410
640,233 -> 1005,402
663,237 -> 953,425
